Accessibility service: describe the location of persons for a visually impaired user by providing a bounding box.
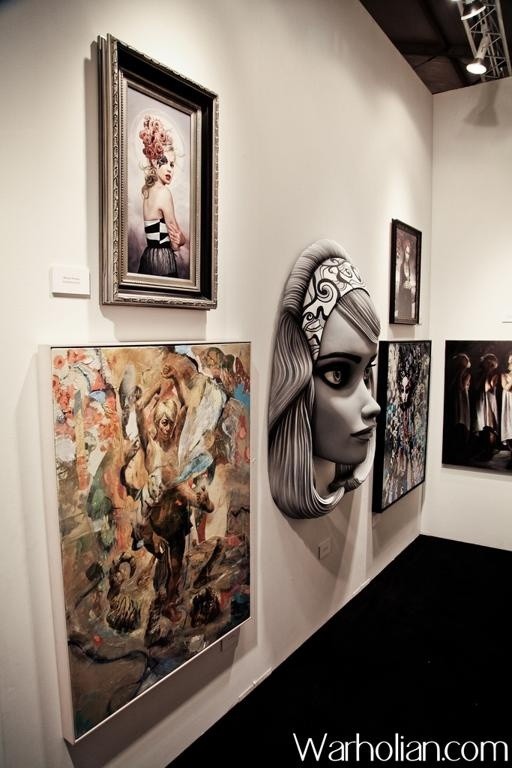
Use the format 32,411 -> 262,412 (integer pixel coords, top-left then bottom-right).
267,237 -> 382,520
500,351 -> 512,452
397,237 -> 417,321
383,342 -> 429,508
133,113 -> 186,279
474,353 -> 500,448
47,344 -> 249,657
448,352 -> 471,432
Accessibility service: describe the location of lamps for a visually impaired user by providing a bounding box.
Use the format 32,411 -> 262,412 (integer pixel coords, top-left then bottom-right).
466,35 -> 491,75
460,0 -> 484,21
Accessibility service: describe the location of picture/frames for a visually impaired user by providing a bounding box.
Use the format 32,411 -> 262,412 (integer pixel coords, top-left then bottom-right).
390,218 -> 423,326
95,32 -> 220,309
